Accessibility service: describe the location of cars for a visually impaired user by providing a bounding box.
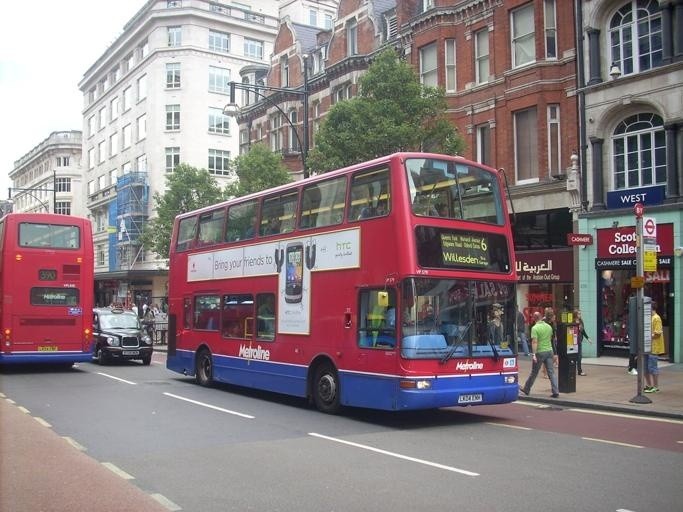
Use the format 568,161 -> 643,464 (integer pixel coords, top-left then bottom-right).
92,308 -> 151,365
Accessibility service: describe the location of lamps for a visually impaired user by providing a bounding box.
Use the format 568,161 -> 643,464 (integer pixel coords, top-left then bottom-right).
608,66 -> 622,81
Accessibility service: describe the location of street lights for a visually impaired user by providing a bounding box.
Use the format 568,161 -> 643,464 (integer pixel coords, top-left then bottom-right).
5,168 -> 57,213
221,80 -> 308,176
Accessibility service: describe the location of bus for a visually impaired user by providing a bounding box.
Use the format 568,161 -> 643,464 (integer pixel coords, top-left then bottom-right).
167,153 -> 518,415
0,215 -> 94,365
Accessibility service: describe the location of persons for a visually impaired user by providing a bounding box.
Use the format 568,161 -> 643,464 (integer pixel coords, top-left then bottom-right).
434,202 -> 451,218
423,305 -> 436,328
626,300 -> 666,393
384,296 -> 415,339
109,299 -> 168,318
369,200 -> 387,217
519,307 -> 592,398
264,215 -> 281,236
440,303 -> 506,346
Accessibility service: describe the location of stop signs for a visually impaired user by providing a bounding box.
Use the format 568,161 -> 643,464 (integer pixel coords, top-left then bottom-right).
643,218 -> 656,238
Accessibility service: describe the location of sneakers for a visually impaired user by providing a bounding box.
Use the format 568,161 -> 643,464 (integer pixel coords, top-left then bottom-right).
628,367 -> 638,376
520,385 -> 529,395
551,393 -> 559,398
578,371 -> 587,377
643,384 -> 659,393
